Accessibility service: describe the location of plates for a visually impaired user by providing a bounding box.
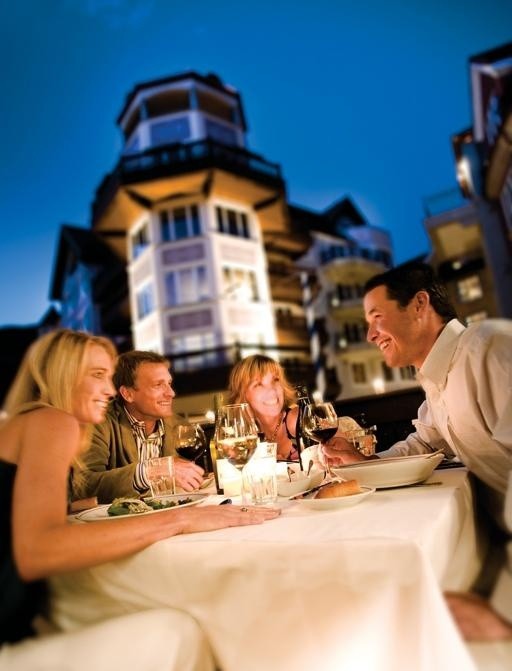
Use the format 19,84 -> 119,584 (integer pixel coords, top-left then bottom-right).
288,485 -> 377,508
74,493 -> 211,522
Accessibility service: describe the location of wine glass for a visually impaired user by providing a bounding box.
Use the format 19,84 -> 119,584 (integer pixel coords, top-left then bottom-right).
174,402 -> 339,489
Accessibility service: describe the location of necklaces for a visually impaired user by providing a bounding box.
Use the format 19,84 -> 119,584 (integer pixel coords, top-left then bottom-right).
263,411 -> 283,442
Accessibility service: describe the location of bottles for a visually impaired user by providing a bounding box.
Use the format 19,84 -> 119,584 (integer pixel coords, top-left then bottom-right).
209,392 -> 225,496
295,384 -> 321,472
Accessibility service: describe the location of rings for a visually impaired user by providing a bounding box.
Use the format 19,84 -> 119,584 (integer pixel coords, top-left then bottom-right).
241,507 -> 248,512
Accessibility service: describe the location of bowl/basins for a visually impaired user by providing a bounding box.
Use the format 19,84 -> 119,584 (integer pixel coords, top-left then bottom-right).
330,453 -> 445,489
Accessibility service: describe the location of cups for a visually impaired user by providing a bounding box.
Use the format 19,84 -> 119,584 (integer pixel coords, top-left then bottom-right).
144,429 -> 375,506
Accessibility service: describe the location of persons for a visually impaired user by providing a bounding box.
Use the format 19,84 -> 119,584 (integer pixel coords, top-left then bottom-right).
227,354 -> 302,464
0,328 -> 282,671
72,350 -> 205,492
315,266 -> 511,645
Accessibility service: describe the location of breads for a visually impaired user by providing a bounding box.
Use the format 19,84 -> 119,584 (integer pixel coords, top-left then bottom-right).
313,478 -> 363,499
